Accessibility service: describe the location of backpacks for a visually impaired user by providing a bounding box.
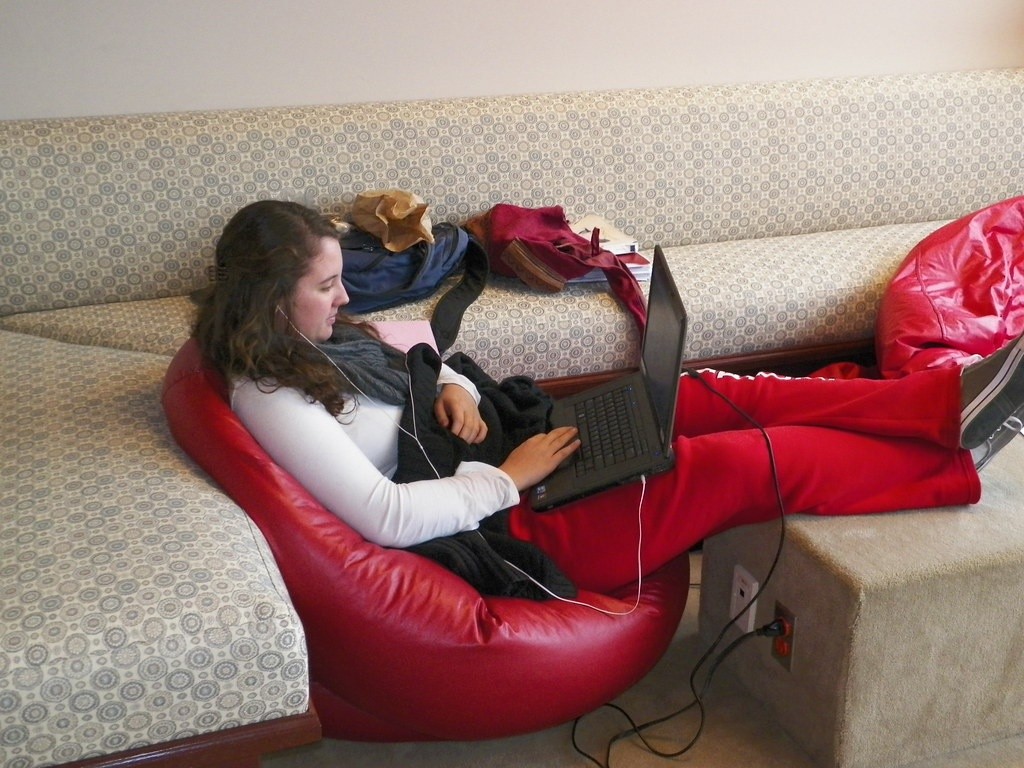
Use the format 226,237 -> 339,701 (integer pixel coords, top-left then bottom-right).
339,222 -> 490,357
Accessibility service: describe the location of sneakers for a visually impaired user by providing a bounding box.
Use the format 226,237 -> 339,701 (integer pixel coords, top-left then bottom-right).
970,403 -> 1024,473
959,331 -> 1024,449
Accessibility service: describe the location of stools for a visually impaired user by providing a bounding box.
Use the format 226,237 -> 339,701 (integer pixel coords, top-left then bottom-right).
699,427 -> 1024,768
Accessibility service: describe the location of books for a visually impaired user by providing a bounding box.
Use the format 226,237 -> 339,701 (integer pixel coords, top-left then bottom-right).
559,214 -> 652,284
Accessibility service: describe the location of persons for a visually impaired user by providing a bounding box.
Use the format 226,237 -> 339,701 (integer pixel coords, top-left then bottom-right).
192,199 -> 1024,601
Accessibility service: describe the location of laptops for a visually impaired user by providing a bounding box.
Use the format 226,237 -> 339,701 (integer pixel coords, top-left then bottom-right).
528,245 -> 689,512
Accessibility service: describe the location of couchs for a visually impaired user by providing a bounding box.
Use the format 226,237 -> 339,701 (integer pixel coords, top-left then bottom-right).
0,69 -> 1023,768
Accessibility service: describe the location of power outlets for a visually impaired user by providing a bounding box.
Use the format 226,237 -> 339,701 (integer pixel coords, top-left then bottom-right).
769,598 -> 796,673
